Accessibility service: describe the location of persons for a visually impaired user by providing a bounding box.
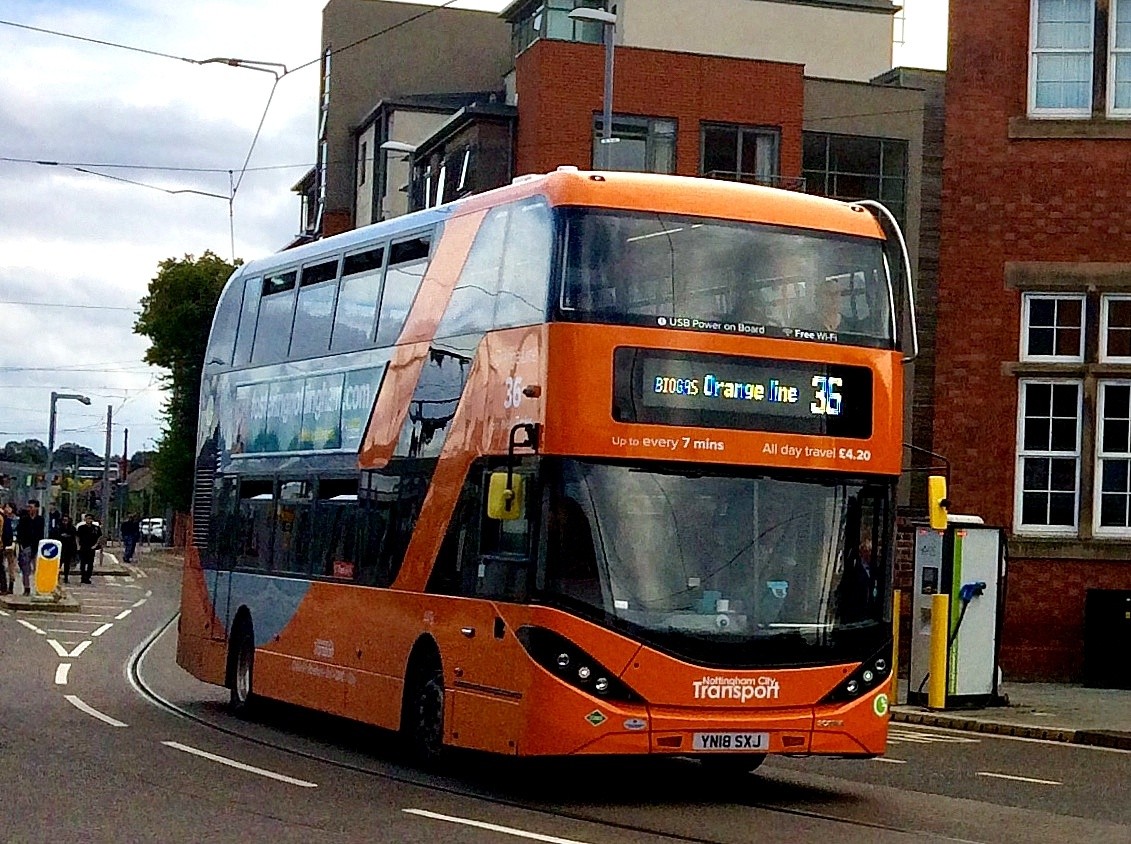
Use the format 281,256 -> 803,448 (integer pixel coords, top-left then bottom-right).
41,501 -> 103,584
0,499 -> 45,597
793,281 -> 861,332
859,540 -> 877,601
121,514 -> 140,563
653,498 -> 795,622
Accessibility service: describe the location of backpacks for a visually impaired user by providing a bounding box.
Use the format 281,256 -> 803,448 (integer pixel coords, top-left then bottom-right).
0,511 -> 13,551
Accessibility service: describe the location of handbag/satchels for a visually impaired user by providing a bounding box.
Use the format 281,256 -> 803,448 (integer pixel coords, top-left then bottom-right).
19,545 -> 31,571
6,542 -> 15,552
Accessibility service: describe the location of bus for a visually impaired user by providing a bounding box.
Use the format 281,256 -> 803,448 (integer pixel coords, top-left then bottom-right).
175,164 -> 952,772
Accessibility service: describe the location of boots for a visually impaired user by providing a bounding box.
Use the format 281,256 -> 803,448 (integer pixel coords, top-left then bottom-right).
7,582 -> 13,594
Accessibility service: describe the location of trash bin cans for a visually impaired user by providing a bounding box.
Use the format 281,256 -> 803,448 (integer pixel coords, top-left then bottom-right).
1085,588 -> 1131,690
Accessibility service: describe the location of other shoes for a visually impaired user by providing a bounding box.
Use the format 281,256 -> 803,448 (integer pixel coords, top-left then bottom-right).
0,590 -> 7,595
123,557 -> 131,563
22,588 -> 30,595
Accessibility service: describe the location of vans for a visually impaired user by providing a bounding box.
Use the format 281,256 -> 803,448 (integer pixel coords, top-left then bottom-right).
138,517 -> 168,540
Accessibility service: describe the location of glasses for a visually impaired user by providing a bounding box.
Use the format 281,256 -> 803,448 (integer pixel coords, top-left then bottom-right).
825,290 -> 842,296
26,505 -> 32,508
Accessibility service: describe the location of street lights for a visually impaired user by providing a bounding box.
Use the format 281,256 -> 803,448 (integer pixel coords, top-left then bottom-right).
44,393 -> 93,536
567,7 -> 619,170
383,140 -> 416,214
